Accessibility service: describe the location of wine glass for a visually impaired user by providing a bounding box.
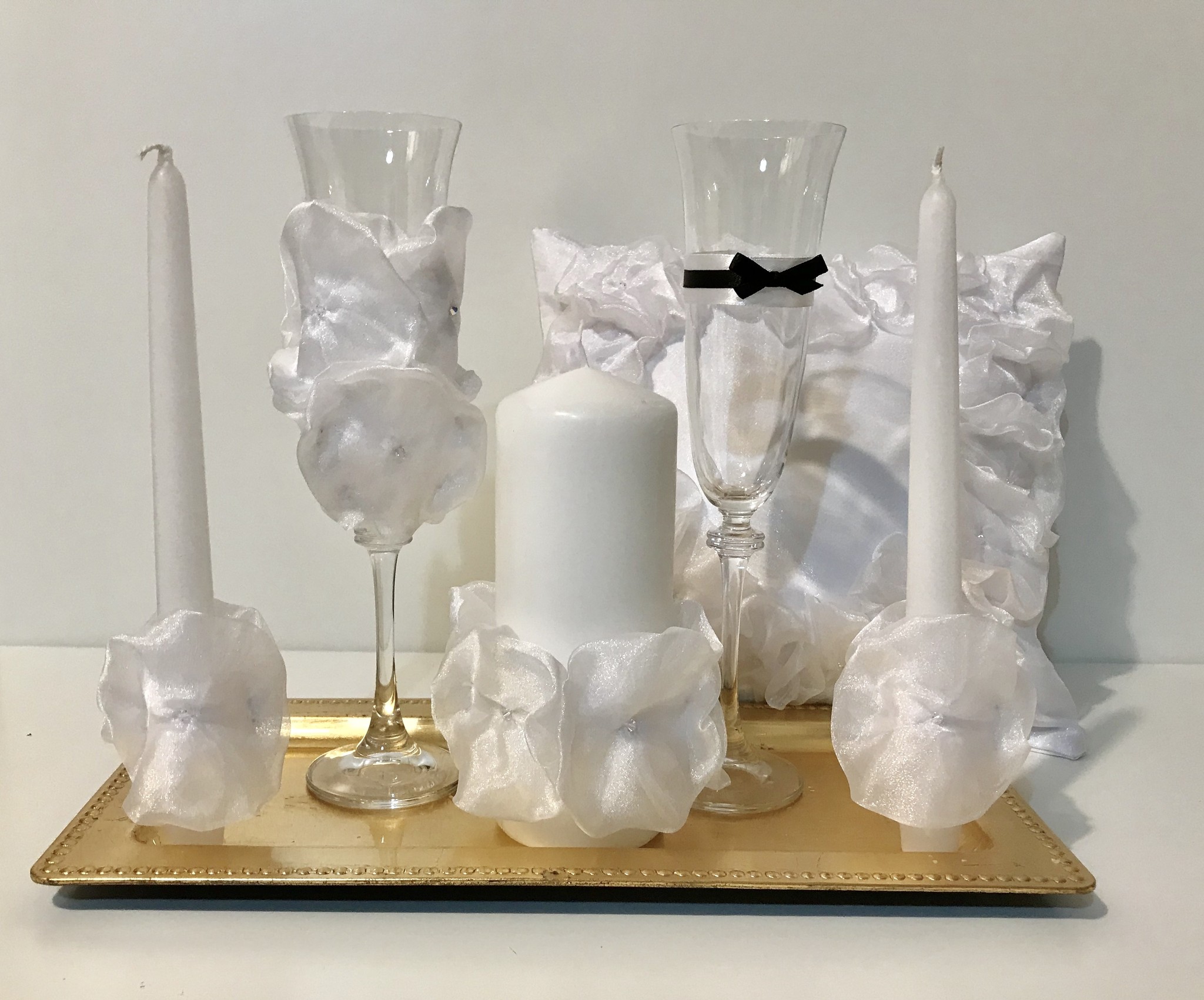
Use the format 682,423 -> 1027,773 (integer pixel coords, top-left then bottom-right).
285,109 -> 487,813
673,117 -> 848,817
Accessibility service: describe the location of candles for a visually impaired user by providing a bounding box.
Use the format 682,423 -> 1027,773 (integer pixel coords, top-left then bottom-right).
138,144 -> 223,847
494,319 -> 678,849
898,142 -> 962,854
